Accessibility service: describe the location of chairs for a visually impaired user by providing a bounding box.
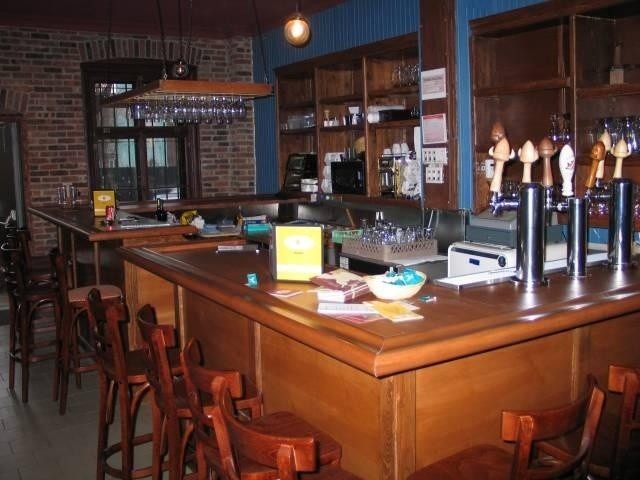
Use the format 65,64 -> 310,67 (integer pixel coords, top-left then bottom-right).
534,365 -> 640,478
406,373 -> 607,480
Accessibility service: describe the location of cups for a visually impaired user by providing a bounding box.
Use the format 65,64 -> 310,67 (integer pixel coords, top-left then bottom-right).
68,184 -> 78,204
57,184 -> 67,204
359,217 -> 432,253
280,114 -> 315,131
391,64 -> 419,88
583,177 -> 640,218
382,141 -> 410,157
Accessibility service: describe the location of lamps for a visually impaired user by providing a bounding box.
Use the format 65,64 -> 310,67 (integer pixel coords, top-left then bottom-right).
282,0 -> 312,48
171,0 -> 191,78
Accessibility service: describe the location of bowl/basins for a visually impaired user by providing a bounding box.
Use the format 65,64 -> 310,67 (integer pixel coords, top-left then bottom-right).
362,270 -> 428,300
183,232 -> 198,240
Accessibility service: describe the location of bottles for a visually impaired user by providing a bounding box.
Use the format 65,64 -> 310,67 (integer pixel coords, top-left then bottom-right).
545,112 -> 570,142
237,205 -> 244,233
155,198 -> 165,220
595,115 -> 640,152
323,118 -> 333,128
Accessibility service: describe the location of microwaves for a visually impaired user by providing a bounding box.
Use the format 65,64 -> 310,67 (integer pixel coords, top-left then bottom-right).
331,162 -> 365,194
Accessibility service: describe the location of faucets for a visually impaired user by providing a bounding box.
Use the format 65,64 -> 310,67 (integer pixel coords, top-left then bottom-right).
156,198 -> 167,221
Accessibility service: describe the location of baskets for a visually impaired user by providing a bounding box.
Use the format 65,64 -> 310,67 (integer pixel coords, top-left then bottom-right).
341,238 -> 438,261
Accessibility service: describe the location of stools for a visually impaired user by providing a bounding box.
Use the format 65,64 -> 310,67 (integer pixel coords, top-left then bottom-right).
135,301 -> 263,479
182,335 -> 346,479
84,286 -> 182,479
1,214 -> 124,413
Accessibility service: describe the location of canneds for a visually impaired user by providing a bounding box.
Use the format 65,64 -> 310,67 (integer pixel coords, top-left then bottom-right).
106,205 -> 115,222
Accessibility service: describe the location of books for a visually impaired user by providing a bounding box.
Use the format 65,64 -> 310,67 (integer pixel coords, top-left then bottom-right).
310,268 -> 370,302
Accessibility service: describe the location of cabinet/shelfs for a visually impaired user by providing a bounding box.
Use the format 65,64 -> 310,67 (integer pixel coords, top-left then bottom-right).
272,31 -> 424,213
468,1 -> 640,230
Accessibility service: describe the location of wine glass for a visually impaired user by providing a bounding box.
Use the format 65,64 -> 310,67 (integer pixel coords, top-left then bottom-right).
129,93 -> 247,123
